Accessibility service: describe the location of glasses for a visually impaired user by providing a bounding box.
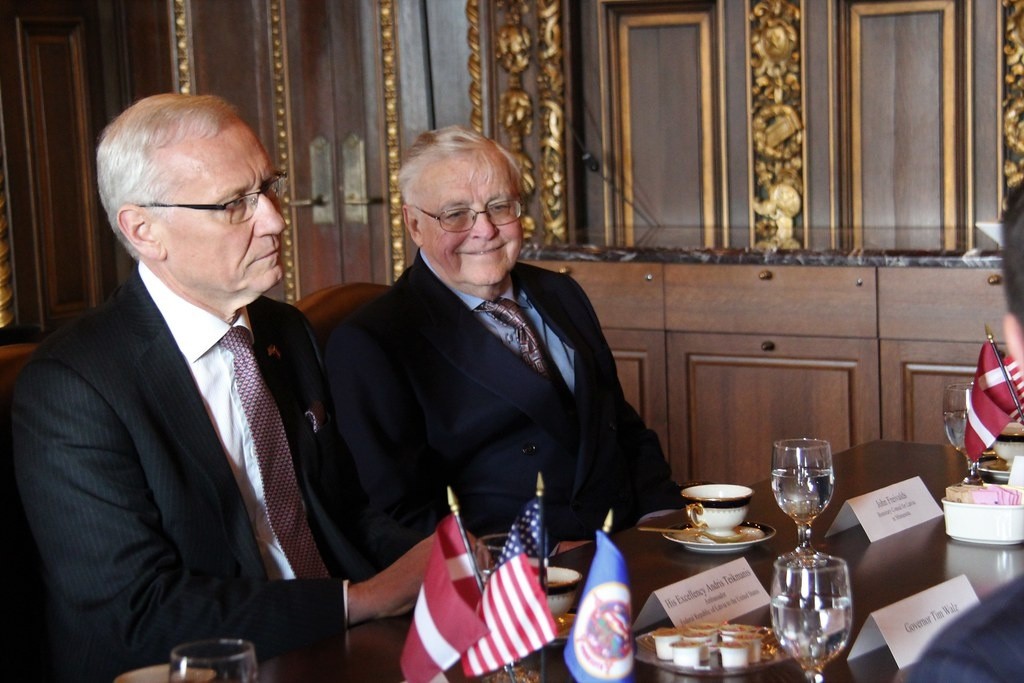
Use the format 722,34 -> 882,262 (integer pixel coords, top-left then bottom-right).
409,198 -> 526,233
136,169 -> 289,225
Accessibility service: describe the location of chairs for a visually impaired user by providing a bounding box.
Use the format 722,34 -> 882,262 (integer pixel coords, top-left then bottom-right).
0,281 -> 387,408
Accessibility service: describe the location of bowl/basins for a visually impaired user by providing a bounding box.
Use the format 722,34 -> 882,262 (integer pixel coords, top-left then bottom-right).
681,485 -> 754,539
546,566 -> 583,628
994,434 -> 1024,466
942,497 -> 1024,545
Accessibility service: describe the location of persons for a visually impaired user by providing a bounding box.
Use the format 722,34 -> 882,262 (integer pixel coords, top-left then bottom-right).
908,179 -> 1024,683
326,126 -> 695,575
0,92 -> 493,683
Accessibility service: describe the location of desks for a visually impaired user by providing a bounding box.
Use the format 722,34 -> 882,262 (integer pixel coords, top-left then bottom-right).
255,436 -> 1024,683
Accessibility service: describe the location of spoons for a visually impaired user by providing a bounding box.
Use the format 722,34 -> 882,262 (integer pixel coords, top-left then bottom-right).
637,527 -> 745,543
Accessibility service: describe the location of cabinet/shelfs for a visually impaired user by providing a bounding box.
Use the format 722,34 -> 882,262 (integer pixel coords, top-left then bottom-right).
517,261 -> 1006,491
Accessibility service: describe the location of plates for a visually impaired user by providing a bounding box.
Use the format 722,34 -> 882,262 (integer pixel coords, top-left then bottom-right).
664,523 -> 776,553
545,613 -> 578,647
978,462 -> 1010,482
635,623 -> 791,675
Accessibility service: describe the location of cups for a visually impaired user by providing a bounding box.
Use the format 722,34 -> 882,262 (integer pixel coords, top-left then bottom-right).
1007,456 -> 1024,488
168,640 -> 260,682
475,533 -> 541,683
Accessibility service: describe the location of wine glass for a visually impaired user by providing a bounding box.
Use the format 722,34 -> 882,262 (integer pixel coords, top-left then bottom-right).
943,382 -> 988,487
771,438 -> 834,569
770,557 -> 853,682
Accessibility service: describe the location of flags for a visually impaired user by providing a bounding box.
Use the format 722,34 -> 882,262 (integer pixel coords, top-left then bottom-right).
564,529 -> 637,683
965,321 -> 1024,462
461,495 -> 559,677
400,513 -> 492,683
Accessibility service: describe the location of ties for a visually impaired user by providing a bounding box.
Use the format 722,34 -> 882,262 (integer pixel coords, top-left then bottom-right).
471,296 -> 574,410
217,329 -> 332,580
